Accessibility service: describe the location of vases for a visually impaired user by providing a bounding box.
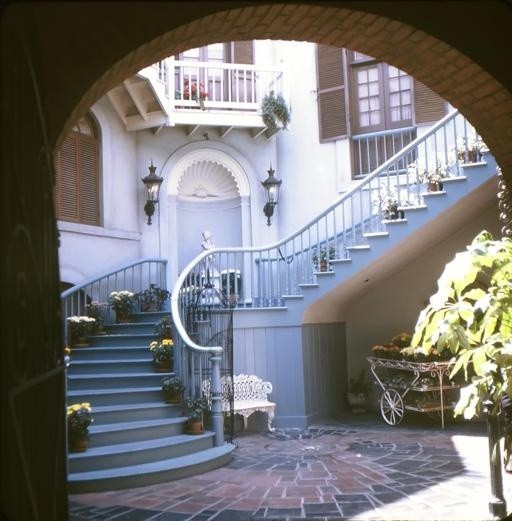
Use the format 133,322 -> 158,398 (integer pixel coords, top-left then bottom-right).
69,430 -> 89,453
153,358 -> 171,372
72,336 -> 90,347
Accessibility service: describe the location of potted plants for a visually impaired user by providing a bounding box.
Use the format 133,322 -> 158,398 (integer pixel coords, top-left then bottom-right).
162,376 -> 186,403
182,397 -> 208,434
221,268 -> 242,305
345,368 -> 374,415
313,245 -> 335,272
135,283 -> 172,312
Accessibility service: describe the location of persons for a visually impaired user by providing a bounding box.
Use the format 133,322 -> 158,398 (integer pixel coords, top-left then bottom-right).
486,340 -> 512,473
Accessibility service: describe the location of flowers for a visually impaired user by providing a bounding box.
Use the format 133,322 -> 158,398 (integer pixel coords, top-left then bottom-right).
370,134 -> 487,222
67,402 -> 94,444
371,332 -> 451,363
183,78 -> 209,100
66,290 -> 136,338
149,339 -> 173,366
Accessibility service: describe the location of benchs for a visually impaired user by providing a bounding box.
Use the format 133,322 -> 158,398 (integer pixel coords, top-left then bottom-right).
202,374 -> 277,432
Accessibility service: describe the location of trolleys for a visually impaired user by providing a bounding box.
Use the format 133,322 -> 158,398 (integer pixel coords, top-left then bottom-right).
366,353 -> 473,429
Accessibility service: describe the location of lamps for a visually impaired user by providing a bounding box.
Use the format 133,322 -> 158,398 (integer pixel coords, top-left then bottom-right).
261,170 -> 283,226
142,159 -> 164,226
